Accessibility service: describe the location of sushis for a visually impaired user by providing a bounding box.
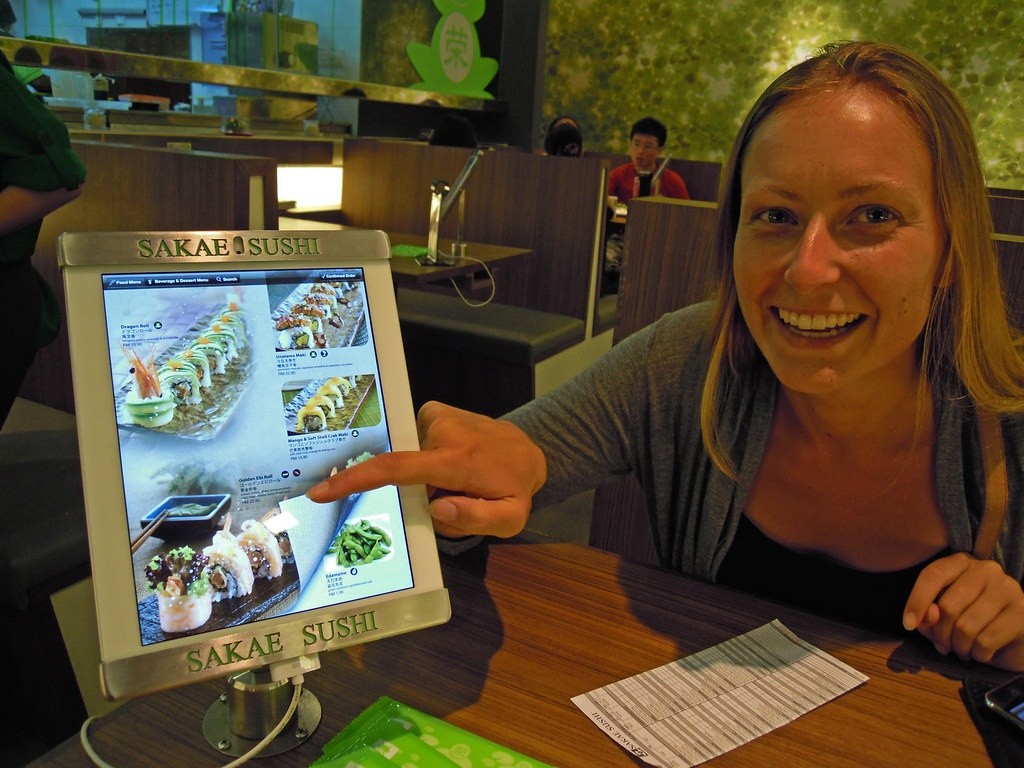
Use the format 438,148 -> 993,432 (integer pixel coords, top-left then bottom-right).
276,282 -> 350,350
145,509 -> 297,634
296,375 -> 361,433
125,298 -> 251,428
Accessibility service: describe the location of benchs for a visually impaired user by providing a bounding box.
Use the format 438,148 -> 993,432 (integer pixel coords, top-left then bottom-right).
0,135 -> 1024,768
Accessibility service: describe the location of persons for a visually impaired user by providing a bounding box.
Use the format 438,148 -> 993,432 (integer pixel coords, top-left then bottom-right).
304,40 -> 1024,674
0,67 -> 87,427
545,117 -> 613,223
430,117 -> 477,149
0,0 -> 51,93
606,117 -> 688,271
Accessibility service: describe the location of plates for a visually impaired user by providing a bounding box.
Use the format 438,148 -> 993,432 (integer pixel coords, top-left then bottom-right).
270,282 -> 367,351
137,514 -> 312,648
139,492 -> 231,544
283,373 -> 377,441
112,302 -> 270,443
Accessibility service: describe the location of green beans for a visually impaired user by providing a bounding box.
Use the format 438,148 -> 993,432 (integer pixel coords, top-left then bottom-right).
325,517 -> 392,567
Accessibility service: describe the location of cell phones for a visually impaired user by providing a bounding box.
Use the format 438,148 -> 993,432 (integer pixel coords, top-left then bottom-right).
986,675 -> 1024,730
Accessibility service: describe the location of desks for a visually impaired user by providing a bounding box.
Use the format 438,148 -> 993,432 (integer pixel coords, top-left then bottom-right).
18,527 -> 1024,767
278,217 -> 535,301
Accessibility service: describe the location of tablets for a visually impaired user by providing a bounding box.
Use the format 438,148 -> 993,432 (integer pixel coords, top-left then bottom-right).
60,229 -> 462,701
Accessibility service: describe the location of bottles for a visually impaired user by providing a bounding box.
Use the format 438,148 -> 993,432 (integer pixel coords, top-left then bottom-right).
75,71 -> 94,101
92,73 -> 109,101
301,118 -> 320,137
84,109 -> 108,130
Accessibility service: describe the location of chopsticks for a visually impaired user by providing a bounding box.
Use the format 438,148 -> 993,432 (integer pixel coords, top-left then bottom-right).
129,507 -> 171,557
